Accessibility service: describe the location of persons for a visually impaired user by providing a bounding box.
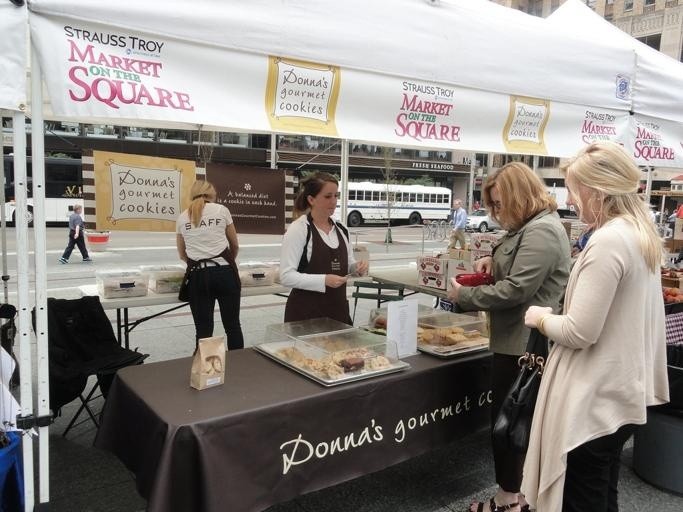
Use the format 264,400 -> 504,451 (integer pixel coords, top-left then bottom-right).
443,159 -> 572,512
276,171 -> 369,328
521,141 -> 672,511
571,225 -> 593,257
58,205 -> 92,264
663,201 -> 682,230
472,200 -> 480,212
175,179 -> 245,357
447,199 -> 468,250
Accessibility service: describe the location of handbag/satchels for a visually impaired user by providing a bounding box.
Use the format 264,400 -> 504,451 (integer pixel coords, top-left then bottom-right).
493,328 -> 548,452
456,273 -> 493,286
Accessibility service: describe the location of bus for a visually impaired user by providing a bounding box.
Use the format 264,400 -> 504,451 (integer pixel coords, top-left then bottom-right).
0,153 -> 84,229
332,181 -> 452,228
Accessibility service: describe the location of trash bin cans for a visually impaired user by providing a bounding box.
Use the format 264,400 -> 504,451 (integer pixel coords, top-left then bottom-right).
633,343 -> 683,499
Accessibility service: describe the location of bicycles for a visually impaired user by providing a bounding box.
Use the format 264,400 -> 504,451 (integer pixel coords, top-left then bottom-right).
420,218 -> 446,242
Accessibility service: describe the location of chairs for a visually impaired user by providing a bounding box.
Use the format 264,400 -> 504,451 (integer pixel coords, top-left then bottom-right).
31,296 -> 149,437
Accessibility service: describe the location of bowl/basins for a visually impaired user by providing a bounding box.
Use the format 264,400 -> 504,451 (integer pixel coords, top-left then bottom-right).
454,273 -> 494,287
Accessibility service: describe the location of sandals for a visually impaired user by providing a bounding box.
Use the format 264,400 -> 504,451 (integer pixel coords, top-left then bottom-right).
470,498 -> 529,512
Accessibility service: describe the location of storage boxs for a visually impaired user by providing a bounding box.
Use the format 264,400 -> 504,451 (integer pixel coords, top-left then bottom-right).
417,232 -> 501,292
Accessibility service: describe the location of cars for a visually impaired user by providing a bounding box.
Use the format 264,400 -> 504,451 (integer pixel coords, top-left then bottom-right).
465,206 -> 494,233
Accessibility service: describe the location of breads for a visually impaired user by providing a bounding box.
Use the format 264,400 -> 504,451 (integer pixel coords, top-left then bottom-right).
277,315 -> 482,382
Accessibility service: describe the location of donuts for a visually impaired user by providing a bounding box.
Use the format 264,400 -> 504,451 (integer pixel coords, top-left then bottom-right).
661,285 -> 683,305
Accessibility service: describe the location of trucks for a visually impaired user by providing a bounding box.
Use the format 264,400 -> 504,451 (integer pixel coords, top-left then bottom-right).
546,186 -> 576,217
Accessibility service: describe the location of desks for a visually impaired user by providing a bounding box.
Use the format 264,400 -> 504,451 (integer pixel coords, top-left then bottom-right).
76,281 -> 292,350
370,269 -> 451,316
92,325 -> 493,512
